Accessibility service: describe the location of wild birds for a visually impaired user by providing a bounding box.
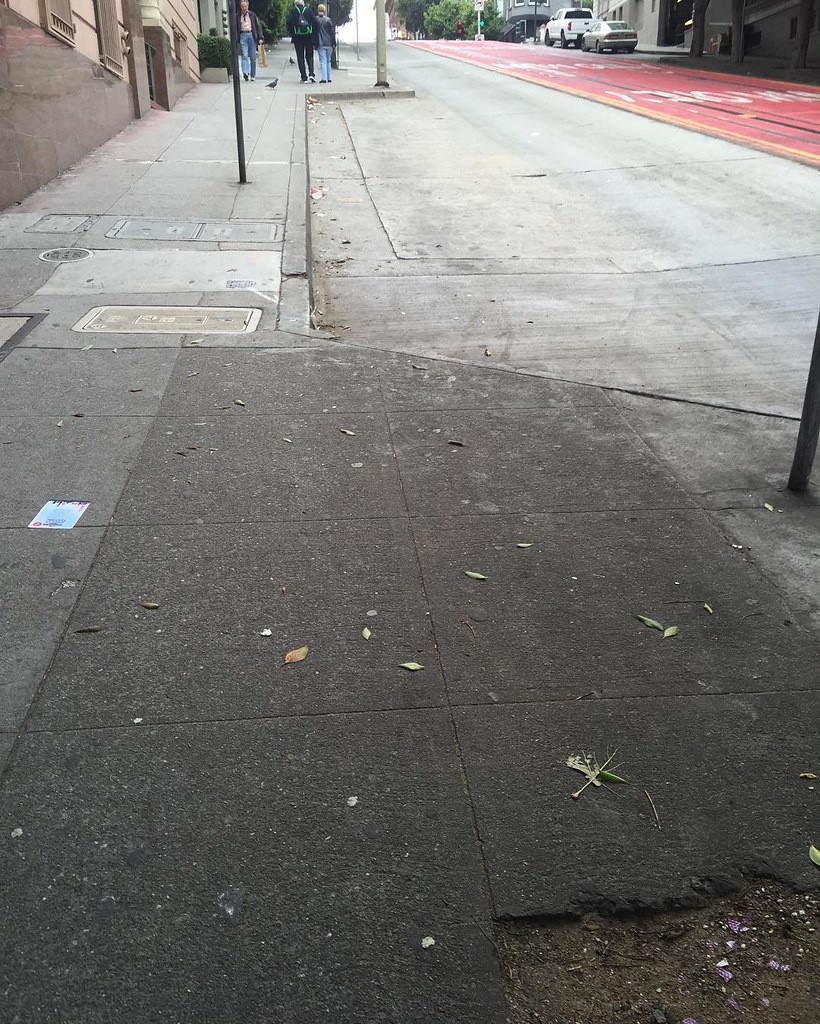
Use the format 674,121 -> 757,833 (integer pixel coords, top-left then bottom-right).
289,57 -> 296,66
266,79 -> 279,89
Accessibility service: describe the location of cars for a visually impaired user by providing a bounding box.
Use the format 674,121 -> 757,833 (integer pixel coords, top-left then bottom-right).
579,20 -> 639,55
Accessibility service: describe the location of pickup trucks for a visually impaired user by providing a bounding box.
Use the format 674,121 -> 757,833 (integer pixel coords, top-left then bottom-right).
544,9 -> 603,49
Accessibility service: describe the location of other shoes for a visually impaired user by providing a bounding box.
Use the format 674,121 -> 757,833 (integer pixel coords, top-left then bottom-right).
328,80 -> 331,82
244,73 -> 249,81
251,76 -> 255,81
310,76 -> 316,82
320,80 -> 326,83
300,78 -> 308,83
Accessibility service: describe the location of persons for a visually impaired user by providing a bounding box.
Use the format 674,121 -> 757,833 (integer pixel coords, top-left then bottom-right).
236,0 -> 264,81
315,3 -> 335,83
286,0 -> 318,84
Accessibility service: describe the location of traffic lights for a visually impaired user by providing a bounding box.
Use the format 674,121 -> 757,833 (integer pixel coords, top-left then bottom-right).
480,13 -> 484,29
457,21 -> 463,35
516,21 -> 521,38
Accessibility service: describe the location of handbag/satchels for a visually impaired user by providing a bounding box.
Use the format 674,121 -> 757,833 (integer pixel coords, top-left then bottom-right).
257,43 -> 268,67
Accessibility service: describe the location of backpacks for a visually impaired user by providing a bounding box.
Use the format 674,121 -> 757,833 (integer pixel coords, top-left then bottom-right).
294,6 -> 312,34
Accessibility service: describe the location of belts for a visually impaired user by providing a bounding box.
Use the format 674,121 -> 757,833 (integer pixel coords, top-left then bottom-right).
240,31 -> 252,33
